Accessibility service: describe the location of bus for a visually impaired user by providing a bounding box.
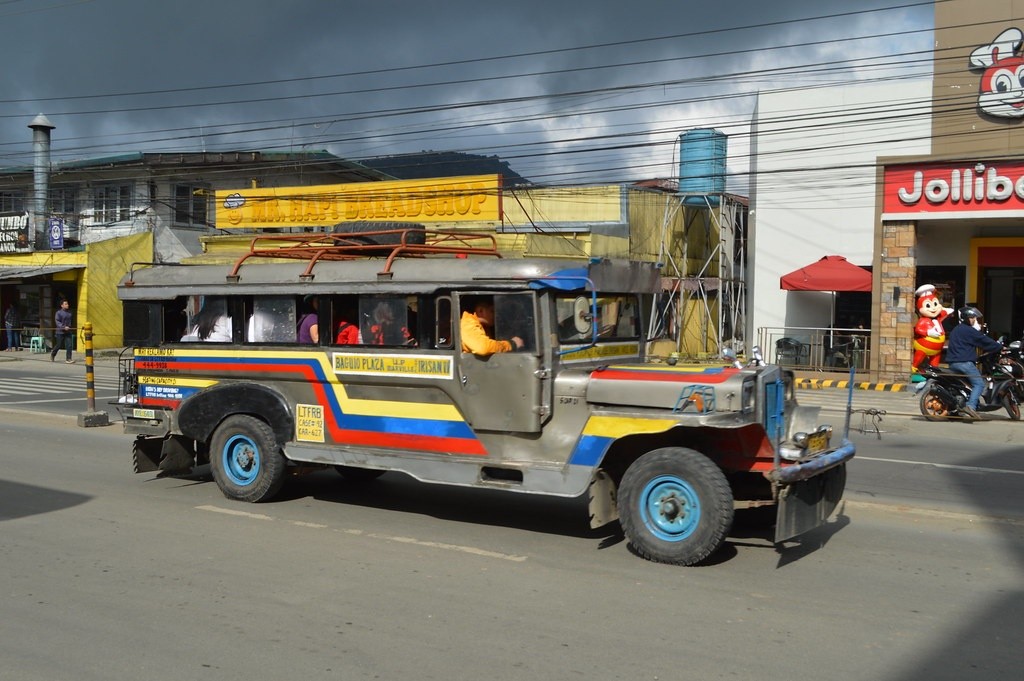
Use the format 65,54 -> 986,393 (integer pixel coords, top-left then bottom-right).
117,228 -> 857,565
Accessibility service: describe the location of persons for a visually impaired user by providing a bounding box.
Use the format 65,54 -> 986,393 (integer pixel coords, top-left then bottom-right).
50,299 -> 75,364
4,301 -> 21,352
459,295 -> 525,355
945,306 -> 1007,420
823,323 -> 851,372
163,294 -> 421,348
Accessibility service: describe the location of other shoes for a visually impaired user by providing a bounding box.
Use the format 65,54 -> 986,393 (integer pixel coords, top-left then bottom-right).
6,349 -> 13,352
14,348 -> 17,350
66,360 -> 75,364
50,354 -> 55,362
963,407 -> 982,421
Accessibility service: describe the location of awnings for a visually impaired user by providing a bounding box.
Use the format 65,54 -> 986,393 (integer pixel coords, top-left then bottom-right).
0,265 -> 87,279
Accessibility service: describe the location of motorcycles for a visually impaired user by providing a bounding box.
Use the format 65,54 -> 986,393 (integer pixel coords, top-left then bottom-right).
994,340 -> 1024,401
911,335 -> 1021,422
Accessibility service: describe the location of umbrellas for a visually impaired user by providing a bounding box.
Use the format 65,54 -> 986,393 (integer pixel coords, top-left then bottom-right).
780,254 -> 873,349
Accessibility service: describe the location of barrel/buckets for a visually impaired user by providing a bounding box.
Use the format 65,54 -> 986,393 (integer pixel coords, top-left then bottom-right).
680,130 -> 728,205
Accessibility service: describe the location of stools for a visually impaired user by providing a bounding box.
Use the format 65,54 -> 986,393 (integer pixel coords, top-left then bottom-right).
30,337 -> 46,354
776,337 -> 811,366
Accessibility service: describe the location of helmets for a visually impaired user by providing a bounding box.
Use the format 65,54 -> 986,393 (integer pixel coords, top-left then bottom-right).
960,306 -> 983,319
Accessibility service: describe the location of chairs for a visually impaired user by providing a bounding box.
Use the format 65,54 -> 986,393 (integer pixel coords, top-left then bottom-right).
822,342 -> 854,373
